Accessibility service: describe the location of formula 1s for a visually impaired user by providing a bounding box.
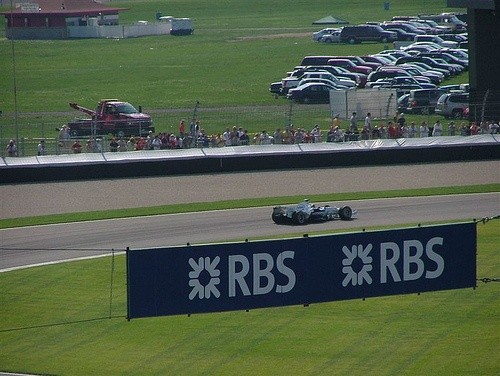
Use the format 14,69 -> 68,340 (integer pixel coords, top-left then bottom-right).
272,199 -> 353,226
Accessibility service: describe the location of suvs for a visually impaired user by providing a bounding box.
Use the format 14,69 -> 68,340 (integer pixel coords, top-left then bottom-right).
435,90 -> 470,120
339,25 -> 398,44
407,88 -> 446,116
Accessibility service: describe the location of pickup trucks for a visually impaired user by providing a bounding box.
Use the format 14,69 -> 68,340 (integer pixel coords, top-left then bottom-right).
67,99 -> 155,140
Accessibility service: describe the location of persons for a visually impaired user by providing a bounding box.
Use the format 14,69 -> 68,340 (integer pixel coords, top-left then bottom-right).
5,138 -> 17,156
311,204 -> 320,208
326,111 -> 500,142
72,118 -> 323,153
37,139 -> 47,155
57,125 -> 70,147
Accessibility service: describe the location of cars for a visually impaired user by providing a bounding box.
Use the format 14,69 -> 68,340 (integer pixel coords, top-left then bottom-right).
268,14 -> 470,114
286,83 -> 343,104
